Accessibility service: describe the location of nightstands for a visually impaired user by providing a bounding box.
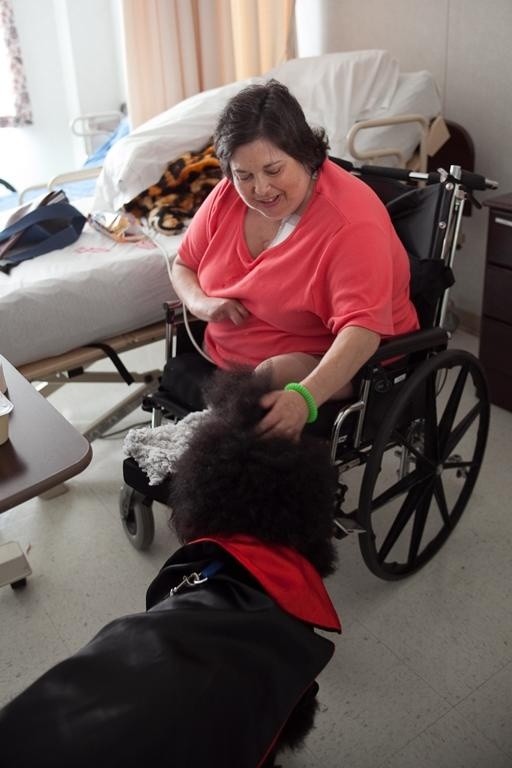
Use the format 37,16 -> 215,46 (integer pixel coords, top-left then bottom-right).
471,193 -> 512,412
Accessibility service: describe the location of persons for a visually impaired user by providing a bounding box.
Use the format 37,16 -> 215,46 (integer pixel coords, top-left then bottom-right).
171,82 -> 420,443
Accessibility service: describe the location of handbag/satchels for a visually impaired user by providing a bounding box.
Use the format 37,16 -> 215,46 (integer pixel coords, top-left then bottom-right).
0,188 -> 88,277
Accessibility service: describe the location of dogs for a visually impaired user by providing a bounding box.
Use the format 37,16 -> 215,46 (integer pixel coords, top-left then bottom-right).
0,362 -> 343,767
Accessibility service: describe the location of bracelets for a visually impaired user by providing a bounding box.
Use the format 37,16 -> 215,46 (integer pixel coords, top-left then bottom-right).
284,383 -> 318,424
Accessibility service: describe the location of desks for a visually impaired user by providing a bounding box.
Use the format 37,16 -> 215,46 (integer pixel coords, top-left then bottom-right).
0,355 -> 96,514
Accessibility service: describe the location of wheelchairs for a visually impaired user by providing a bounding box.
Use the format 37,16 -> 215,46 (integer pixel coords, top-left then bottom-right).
119,156 -> 500,582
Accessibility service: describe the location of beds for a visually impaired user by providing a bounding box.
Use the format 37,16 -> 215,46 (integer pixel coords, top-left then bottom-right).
0,120 -> 477,398
